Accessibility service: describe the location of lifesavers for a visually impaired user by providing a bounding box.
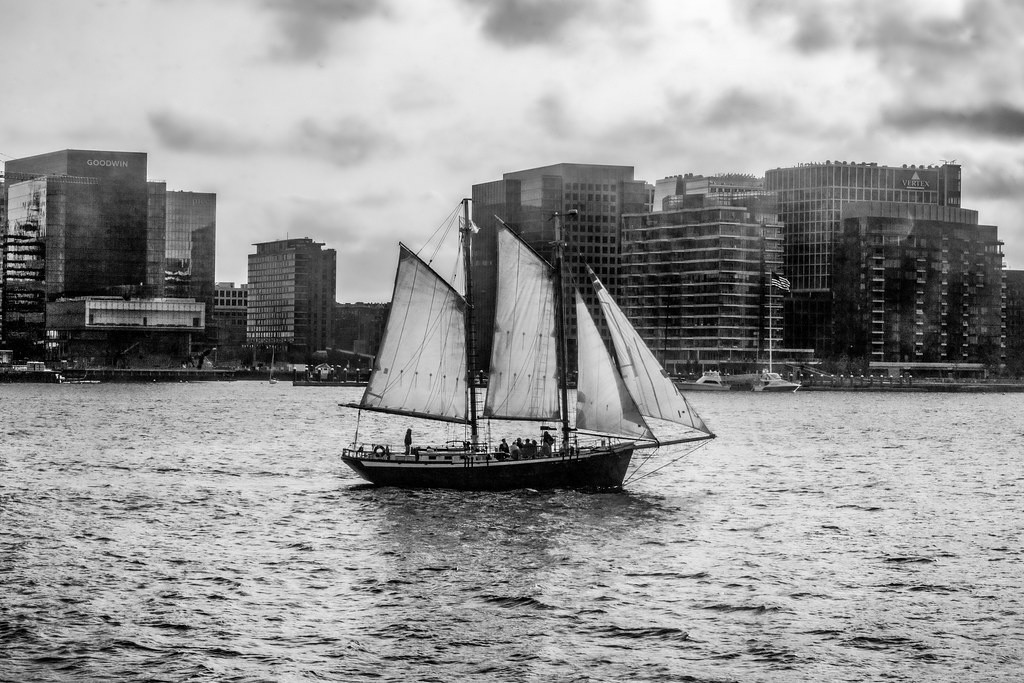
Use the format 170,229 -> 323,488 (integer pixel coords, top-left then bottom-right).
374,445 -> 385,457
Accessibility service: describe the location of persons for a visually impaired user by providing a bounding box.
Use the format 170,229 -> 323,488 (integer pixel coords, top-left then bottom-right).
498,431 -> 554,459
404,428 -> 412,455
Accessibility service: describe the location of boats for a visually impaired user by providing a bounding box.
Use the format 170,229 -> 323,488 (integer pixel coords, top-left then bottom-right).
673,370 -> 732,391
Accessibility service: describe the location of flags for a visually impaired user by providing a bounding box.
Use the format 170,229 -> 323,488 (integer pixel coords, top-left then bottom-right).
771,270 -> 791,292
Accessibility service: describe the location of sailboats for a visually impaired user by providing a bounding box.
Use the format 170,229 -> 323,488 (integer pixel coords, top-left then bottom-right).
269,347 -> 278,384
340,196 -> 719,492
751,268 -> 803,393
